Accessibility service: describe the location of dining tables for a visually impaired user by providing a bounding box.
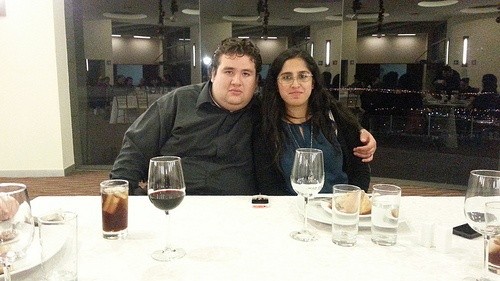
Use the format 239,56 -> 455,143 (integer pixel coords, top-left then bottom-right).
0,195 -> 500,281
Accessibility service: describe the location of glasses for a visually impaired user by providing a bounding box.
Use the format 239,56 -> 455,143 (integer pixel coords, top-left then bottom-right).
277,72 -> 314,83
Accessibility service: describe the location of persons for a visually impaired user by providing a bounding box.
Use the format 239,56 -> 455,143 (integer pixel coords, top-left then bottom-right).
109,36 -> 377,196
252,48 -> 372,194
322,65 -> 500,109
98,75 -> 182,88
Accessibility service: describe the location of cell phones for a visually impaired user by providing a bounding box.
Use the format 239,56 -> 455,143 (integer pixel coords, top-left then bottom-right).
452,224 -> 482,239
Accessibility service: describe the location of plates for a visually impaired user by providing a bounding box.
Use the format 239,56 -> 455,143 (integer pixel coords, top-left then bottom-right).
322,200 -> 370,218
299,199 -> 371,228
0,221 -> 66,279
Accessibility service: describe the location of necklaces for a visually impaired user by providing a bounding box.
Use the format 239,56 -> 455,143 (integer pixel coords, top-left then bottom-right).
286,122 -> 313,179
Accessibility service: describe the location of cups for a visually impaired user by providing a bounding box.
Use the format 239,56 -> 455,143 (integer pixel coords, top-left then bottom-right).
371,184 -> 401,246
332,184 -> 360,245
98,179 -> 129,240
484,201 -> 500,275
36,210 -> 78,281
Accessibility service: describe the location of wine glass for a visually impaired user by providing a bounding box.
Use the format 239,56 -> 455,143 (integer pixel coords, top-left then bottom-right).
291,148 -> 326,241
464,169 -> 500,281
147,156 -> 188,261
0,183 -> 36,281
